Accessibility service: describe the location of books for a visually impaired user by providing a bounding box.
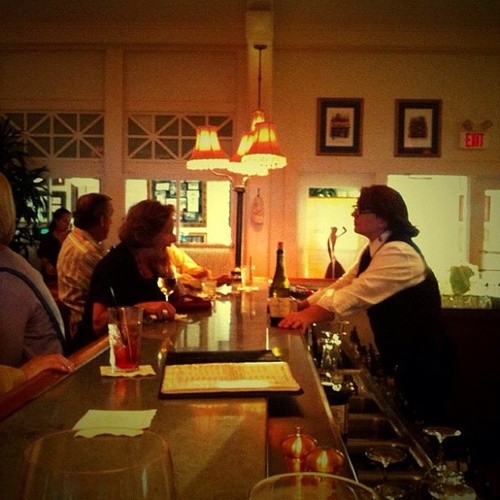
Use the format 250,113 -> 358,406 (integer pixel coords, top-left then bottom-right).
158,349 -> 305,401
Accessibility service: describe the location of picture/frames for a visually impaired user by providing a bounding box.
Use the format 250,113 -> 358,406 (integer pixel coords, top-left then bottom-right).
393,99 -> 442,158
315,97 -> 364,157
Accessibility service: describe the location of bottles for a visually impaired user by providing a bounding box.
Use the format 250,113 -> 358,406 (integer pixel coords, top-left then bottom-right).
267,241 -> 292,327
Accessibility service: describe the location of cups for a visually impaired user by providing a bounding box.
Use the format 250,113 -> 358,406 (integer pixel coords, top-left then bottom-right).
106,305 -> 145,373
248,472 -> 378,500
199,280 -> 218,299
241,267 -> 255,290
22,427 -> 177,500
441,294 -> 494,308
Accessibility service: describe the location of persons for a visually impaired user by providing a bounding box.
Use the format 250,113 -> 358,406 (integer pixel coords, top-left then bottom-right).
64,200 -> 186,358
37,208 -> 71,282
0,353 -> 75,394
278,185 -> 453,426
57,193 -> 114,341
156,242 -> 208,290
0,174 -> 65,369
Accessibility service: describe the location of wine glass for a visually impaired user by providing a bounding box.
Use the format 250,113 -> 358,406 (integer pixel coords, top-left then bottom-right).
417,423 -> 479,500
362,441 -> 415,500
158,265 -> 177,301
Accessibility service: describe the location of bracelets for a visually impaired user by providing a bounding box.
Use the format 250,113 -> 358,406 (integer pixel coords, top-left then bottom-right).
164,276 -> 177,287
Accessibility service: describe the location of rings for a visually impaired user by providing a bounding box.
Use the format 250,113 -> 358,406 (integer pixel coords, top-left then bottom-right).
162,309 -> 168,316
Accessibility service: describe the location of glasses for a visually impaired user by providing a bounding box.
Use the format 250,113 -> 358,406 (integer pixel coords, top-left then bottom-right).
352,204 -> 373,214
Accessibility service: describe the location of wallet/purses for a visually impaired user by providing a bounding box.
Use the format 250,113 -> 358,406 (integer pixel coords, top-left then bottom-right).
173,293 -> 211,311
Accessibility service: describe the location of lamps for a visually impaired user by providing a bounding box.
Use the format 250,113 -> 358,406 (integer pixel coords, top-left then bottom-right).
235,43 -> 269,154
459,118 -> 493,150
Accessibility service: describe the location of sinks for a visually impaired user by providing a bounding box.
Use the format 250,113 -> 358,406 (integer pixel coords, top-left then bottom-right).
346,394 -> 384,416
344,414 -> 404,441
358,476 -> 435,500
347,444 -> 423,471
